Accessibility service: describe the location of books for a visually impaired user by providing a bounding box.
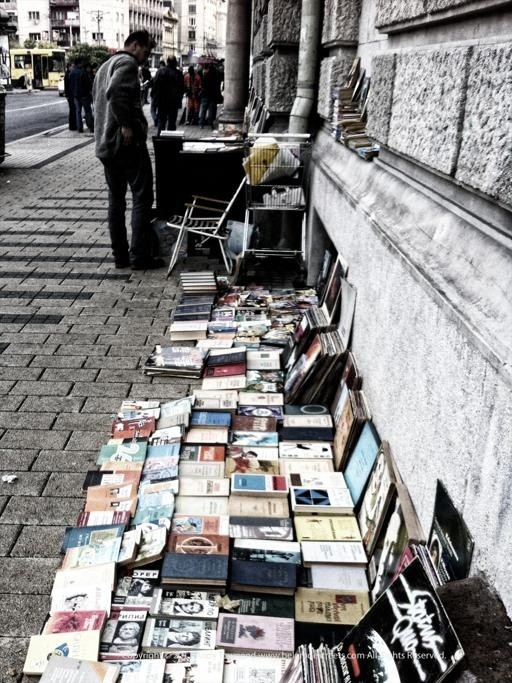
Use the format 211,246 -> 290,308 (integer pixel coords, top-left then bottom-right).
330,56 -> 382,161
159,124 -> 248,154
22,271 -> 477,683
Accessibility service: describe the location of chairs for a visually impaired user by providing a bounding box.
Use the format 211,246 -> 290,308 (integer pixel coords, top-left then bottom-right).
167,175 -> 247,277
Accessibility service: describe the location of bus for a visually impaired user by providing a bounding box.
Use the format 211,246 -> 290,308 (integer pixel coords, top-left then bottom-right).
9,48 -> 66,90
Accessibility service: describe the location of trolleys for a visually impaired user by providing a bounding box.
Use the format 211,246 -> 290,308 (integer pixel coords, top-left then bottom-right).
240,132 -> 312,286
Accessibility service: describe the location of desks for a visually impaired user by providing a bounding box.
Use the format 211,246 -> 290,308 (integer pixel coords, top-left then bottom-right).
152,134 -> 251,221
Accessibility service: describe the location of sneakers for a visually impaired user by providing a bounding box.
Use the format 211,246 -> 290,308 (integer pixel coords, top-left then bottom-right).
115,256 -> 166,270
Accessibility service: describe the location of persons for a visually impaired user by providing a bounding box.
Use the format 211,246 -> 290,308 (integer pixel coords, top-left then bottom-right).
141,55 -> 225,134
65,60 -> 98,133
92,31 -> 165,269
